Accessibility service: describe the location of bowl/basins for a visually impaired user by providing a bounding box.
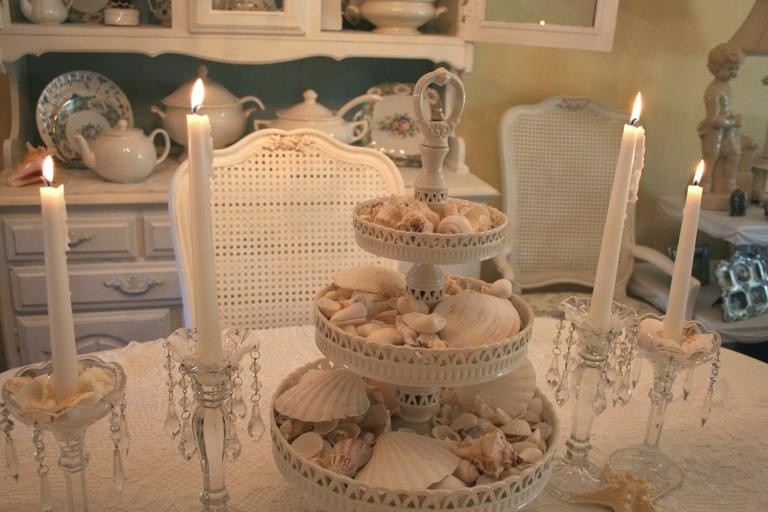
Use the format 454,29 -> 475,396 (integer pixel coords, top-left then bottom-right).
103,9 -> 141,27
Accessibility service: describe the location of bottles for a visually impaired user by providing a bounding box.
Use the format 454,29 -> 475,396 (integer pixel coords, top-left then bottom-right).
729,191 -> 749,216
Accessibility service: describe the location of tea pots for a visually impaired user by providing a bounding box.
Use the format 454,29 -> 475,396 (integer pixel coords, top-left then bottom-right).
74,118 -> 171,183
20,1 -> 76,25
151,64 -> 264,164
255,86 -> 368,146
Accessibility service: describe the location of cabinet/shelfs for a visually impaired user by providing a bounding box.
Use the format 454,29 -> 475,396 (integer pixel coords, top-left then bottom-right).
0,0 -> 189,65
0,202 -> 500,372
630,196 -> 768,343
189,1 -> 620,73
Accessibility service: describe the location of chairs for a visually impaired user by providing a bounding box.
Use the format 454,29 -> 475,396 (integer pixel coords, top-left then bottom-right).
497,96 -> 701,322
168,128 -> 405,331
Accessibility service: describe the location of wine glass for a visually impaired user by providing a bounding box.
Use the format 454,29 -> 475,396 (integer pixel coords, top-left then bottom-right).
358,1 -> 437,35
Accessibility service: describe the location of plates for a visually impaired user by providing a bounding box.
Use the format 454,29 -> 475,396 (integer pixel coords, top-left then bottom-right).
368,83 -> 444,167
37,68 -> 133,167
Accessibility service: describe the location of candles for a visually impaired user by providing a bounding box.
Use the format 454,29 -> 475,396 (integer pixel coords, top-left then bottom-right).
584,92 -> 642,332
185,79 -> 226,365
39,154 -> 80,403
664,158 -> 703,343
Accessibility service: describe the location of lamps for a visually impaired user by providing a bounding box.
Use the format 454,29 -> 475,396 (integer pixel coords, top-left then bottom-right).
728,0 -> 768,205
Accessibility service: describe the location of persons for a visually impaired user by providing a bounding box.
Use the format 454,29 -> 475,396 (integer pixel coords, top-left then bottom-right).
698,42 -> 746,194
